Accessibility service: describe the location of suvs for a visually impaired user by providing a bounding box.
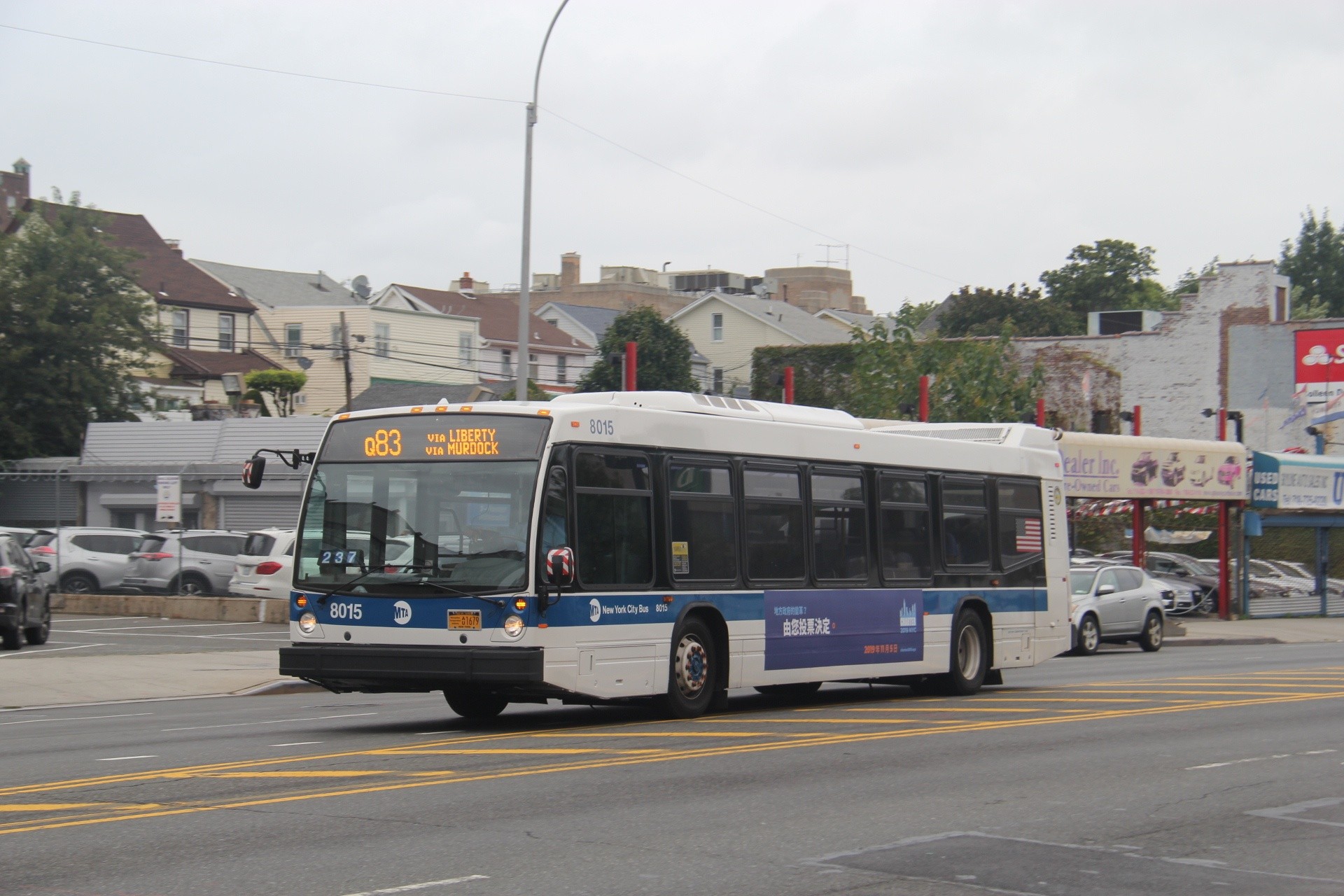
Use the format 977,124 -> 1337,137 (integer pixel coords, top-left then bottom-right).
120,529 -> 251,594
25,525 -> 158,590
361,534 -> 474,575
229,527 -> 392,596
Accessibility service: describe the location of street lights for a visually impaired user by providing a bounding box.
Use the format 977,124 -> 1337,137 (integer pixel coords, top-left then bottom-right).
312,310 -> 356,413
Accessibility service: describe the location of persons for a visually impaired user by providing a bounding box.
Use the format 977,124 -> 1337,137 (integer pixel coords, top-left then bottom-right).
514,492 -> 567,586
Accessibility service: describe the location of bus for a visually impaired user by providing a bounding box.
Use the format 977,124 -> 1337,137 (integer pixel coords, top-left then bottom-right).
242,387 -> 1074,715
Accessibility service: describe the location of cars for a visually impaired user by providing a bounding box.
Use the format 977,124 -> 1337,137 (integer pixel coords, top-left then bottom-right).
0,536 -> 55,650
1066,557 -> 1189,648
0,526 -> 42,545
1068,545 -> 1344,614
